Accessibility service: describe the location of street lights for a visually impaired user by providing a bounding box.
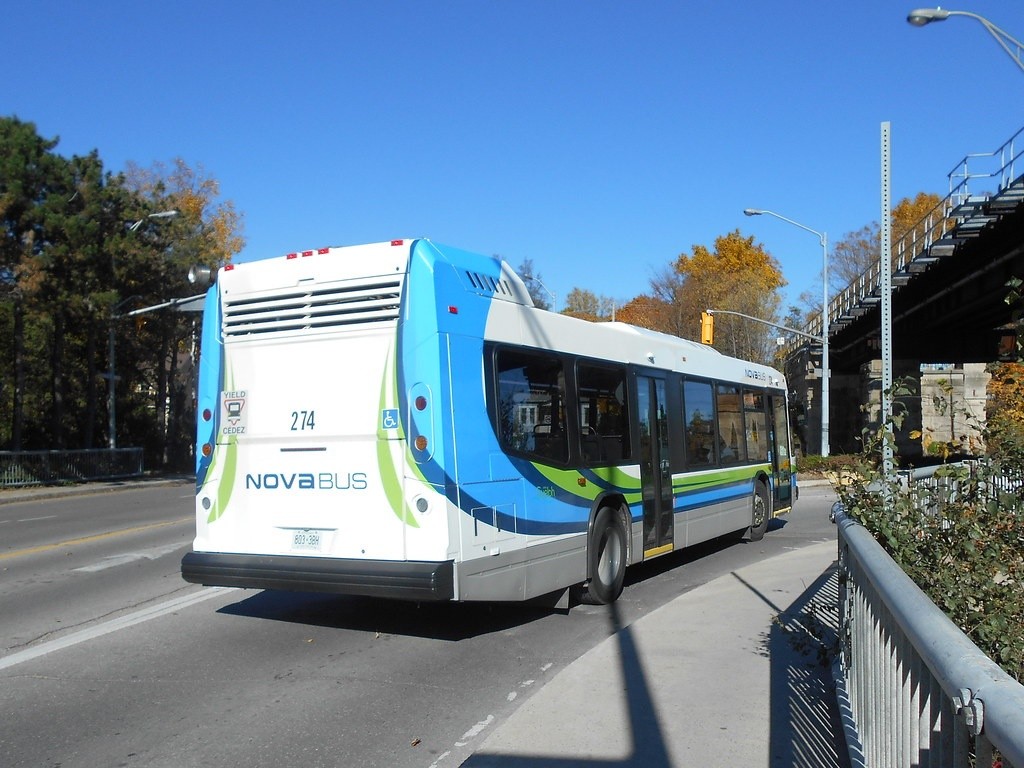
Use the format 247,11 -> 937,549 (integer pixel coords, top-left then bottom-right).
742,209 -> 831,458
525,274 -> 556,313
908,7 -> 1023,72
109,210 -> 179,448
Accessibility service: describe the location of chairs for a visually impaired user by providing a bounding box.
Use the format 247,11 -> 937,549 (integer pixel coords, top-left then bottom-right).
530,424 -> 564,456
580,426 -> 602,462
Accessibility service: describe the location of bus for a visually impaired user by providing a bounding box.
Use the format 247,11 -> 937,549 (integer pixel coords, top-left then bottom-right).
181,237 -> 799,609
